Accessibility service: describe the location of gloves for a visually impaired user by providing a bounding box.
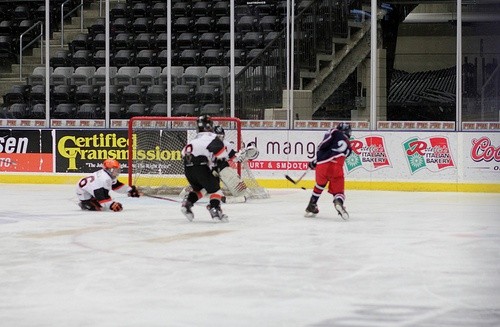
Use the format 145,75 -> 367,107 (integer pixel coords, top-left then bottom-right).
111,201 -> 123,211
308,159 -> 316,168
130,185 -> 140,197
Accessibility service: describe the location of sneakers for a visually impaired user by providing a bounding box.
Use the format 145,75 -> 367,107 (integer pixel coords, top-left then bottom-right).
304,203 -> 319,217
207,205 -> 228,221
333,197 -> 350,219
180,200 -> 194,219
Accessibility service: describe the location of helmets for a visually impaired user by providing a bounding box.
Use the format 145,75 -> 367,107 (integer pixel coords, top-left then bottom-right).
104,158 -> 120,168
198,114 -> 213,131
335,121 -> 352,136
214,126 -> 225,139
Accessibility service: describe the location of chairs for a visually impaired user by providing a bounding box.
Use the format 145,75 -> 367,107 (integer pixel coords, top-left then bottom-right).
0,0 -> 358,118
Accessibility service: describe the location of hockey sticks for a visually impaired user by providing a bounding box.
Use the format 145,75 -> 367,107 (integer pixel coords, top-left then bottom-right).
138,192 -> 209,207
285,167 -> 312,184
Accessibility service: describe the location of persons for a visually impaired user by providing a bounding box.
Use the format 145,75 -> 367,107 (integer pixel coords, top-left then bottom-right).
76,157 -> 140,212
305,124 -> 352,219
179,115 -> 249,221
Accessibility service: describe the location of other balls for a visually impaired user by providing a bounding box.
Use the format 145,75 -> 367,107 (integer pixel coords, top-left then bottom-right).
301,187 -> 306,190
